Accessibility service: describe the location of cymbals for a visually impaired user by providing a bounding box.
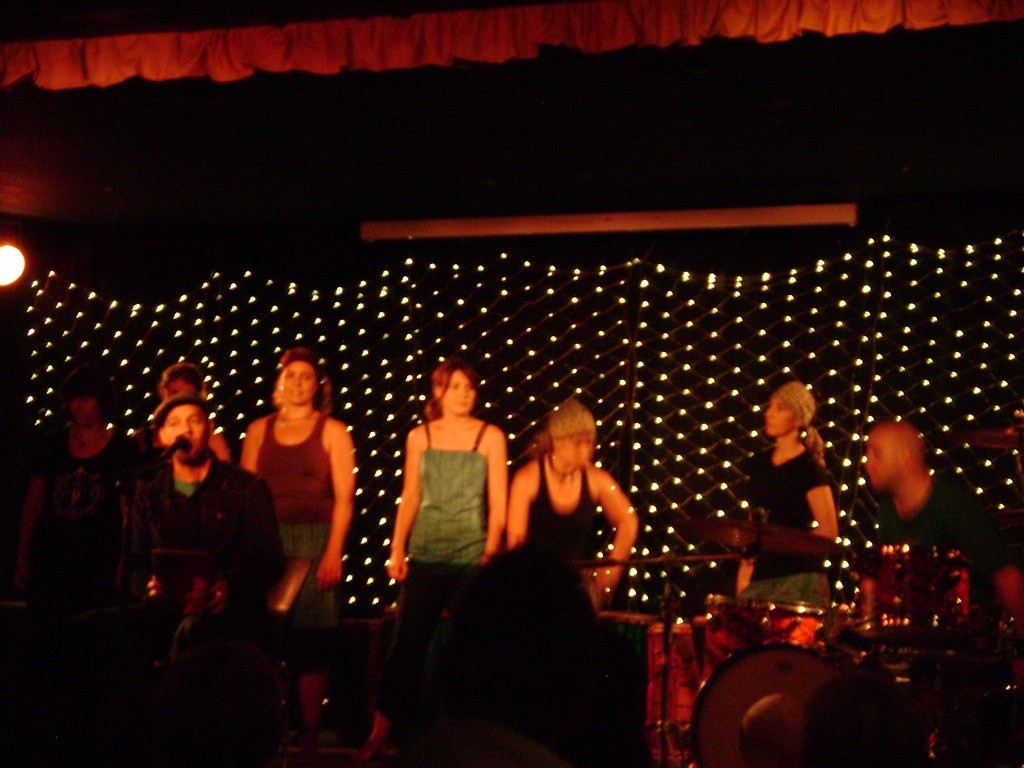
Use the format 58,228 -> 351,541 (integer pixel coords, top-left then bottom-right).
670,515 -> 843,558
575,551 -> 754,566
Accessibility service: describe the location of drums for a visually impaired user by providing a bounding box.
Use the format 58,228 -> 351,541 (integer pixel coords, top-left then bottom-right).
848,539 -> 970,646
689,639 -> 863,767
699,593 -> 830,697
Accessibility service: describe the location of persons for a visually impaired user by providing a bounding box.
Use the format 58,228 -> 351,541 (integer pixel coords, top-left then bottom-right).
15,365 -> 144,768
453,396 -> 642,727
118,398 -> 287,768
126,356 -> 234,467
377,364 -> 511,737
862,412 -> 1024,658
737,378 -> 844,621
230,344 -> 356,768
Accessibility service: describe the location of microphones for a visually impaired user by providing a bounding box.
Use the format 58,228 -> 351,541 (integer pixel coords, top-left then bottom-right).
747,508 -> 766,549
174,434 -> 193,452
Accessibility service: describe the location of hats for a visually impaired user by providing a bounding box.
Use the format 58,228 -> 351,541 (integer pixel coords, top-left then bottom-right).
150,392 -> 211,428
